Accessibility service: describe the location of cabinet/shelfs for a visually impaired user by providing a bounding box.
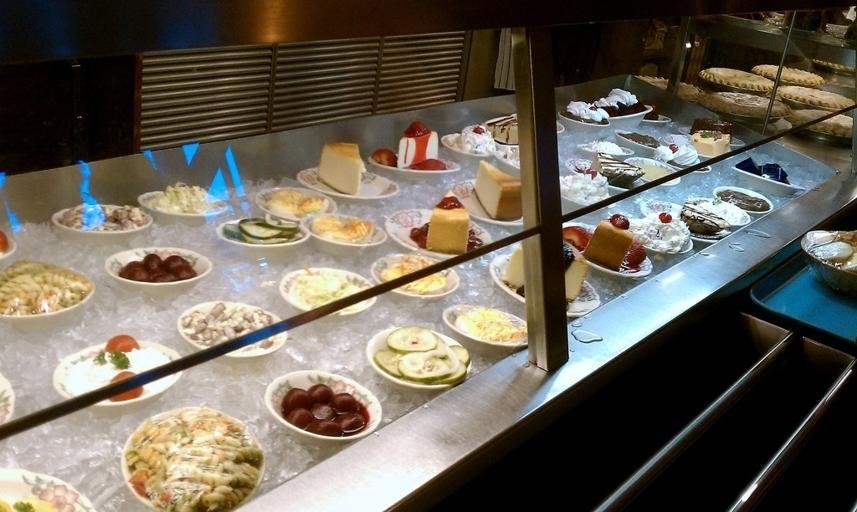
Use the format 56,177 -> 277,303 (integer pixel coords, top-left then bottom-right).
1,1 -> 854,507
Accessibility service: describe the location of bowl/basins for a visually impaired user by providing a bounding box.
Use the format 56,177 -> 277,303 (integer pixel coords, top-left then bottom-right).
798,229 -> 856,304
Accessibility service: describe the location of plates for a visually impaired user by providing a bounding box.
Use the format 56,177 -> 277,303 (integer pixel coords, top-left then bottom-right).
137,189 -> 230,225
279,267 -> 378,318
384,207 -> 494,256
180,300 -> 288,360
483,113 -> 566,146
103,246 -> 213,297
685,195 -> 751,228
439,132 -> 512,161
576,140 -> 635,157
371,252 -> 459,304
612,102 -> 655,120
367,150 -> 463,176
713,184 -> 774,215
489,254 -> 602,317
615,127 -> 662,151
264,368 -> 382,443
568,156 -> 647,190
217,215 -> 310,251
438,301 -> 530,350
366,323 -> 475,392
609,212 -> 696,256
641,198 -> 727,244
624,154 -> 682,187
729,165 -> 806,192
643,112 -> 673,125
52,341 -> 184,410
298,166 -> 401,200
444,179 -> 526,226
665,132 -> 735,160
562,221 -> 652,280
256,186 -> 331,219
654,149 -> 714,173
679,126 -> 747,148
559,107 -> 612,129
52,201 -> 155,241
299,214 -> 387,250
121,405 -> 268,512
1,261 -> 94,327
559,174 -> 618,210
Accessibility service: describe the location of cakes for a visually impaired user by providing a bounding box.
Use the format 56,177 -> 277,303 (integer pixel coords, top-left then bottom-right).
628,64 -> 854,149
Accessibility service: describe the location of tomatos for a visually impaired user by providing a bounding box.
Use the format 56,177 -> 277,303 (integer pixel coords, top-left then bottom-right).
104,334 -> 141,356
110,370 -> 144,401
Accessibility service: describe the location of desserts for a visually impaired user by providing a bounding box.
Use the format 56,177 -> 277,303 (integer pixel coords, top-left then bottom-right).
581,213 -> 648,274
311,213 -> 376,246
557,130 -> 732,207
441,110 -> 522,172
559,88 -> 660,128
473,160 -> 522,222
299,270 -> 351,311
617,156 -> 793,258
318,140 -> 366,196
409,189 -> 485,255
799,225 -> 857,294
502,232 -> 588,303
371,121 -> 445,171
380,255 -> 448,295
